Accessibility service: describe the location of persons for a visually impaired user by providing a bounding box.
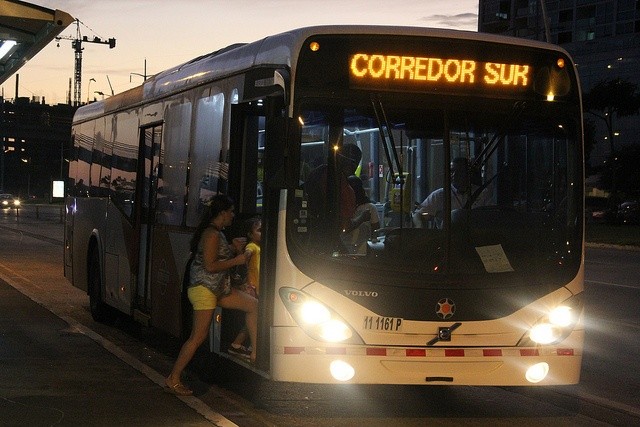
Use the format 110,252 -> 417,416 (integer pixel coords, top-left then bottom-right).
412,155 -> 497,229
228,214 -> 262,357
308,143 -> 371,251
157,193 -> 259,396
345,173 -> 380,242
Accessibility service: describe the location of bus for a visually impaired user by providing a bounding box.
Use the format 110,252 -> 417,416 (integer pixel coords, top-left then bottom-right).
58,22 -> 621,407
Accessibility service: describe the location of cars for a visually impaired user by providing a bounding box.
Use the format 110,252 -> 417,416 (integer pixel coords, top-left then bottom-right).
0,193 -> 20,208
591,201 -> 639,224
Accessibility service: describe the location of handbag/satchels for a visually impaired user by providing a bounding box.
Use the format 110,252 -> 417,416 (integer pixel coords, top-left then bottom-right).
230,263 -> 248,286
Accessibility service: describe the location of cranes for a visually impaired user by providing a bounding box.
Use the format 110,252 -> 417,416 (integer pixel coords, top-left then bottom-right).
54,17 -> 116,106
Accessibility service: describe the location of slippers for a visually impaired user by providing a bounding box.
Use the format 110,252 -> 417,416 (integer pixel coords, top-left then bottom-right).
159,383 -> 192,395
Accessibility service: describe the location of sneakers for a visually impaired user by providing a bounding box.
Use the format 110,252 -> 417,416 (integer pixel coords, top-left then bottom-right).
227,344 -> 250,358
